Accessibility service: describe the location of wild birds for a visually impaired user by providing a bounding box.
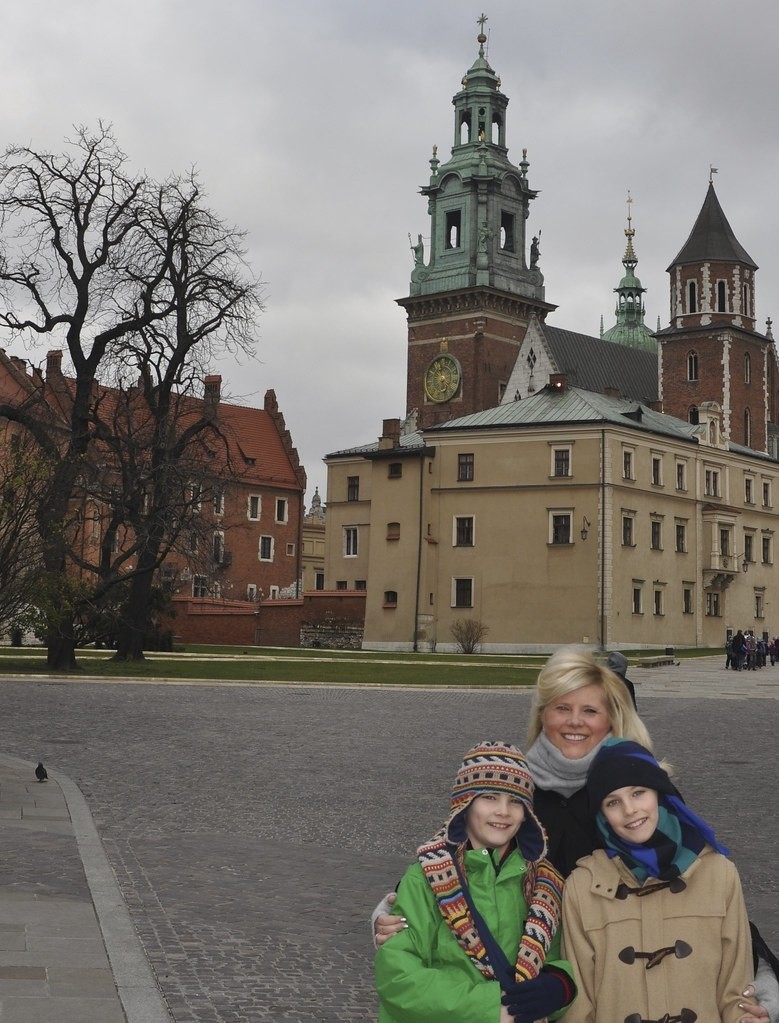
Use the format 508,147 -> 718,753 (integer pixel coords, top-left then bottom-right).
34,762 -> 48,782
514,388 -> 522,401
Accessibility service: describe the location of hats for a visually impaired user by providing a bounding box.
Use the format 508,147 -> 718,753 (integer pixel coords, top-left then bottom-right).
443,740 -> 548,904
584,735 -> 685,821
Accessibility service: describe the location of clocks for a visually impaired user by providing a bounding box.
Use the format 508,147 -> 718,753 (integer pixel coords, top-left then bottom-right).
424,353 -> 462,403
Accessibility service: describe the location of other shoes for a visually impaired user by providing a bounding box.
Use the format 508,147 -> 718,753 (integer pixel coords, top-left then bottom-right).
725,664 -> 774,672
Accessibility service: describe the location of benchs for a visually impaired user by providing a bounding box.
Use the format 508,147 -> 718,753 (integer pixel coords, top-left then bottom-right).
639,655 -> 675,668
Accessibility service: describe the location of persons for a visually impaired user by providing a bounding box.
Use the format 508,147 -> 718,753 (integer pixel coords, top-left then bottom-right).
374,739 -> 580,1023
530,237 -> 540,267
410,234 -> 424,264
725,630 -> 779,672
559,739 -> 758,1023
605,651 -> 637,710
370,644 -> 779,1023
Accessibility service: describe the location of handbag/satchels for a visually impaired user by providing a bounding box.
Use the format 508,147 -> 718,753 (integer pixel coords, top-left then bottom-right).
741,644 -> 746,652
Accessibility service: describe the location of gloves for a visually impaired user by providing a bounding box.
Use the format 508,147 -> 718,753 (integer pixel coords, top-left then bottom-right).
500,967 -> 573,1023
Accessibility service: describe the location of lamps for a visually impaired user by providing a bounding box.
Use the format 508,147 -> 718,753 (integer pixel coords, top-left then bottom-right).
738,550 -> 749,574
580,515 -> 591,542
